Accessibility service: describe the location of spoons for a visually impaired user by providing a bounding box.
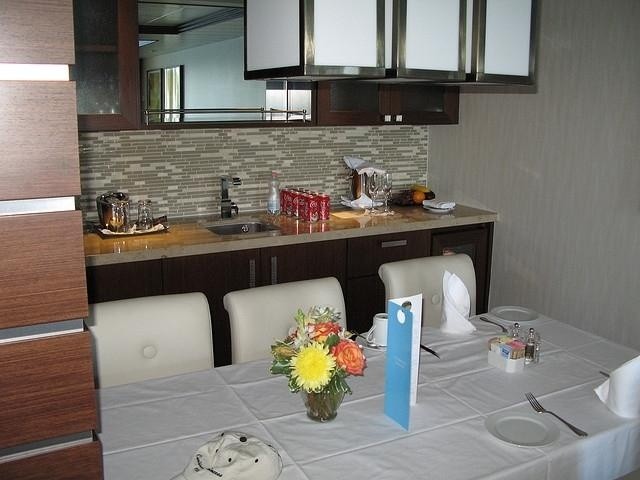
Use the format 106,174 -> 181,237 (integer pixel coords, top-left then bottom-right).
351,330 -> 381,349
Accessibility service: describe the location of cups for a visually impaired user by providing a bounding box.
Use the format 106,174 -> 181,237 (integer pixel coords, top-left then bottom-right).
112,201 -> 129,233
367,313 -> 387,347
138,199 -> 154,230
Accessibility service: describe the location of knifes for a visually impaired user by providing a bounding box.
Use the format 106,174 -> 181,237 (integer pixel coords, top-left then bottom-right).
420,341 -> 442,361
599,369 -> 609,379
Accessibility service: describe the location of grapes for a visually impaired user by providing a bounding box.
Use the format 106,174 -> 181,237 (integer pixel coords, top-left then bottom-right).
392,189 -> 414,205
425,191 -> 436,200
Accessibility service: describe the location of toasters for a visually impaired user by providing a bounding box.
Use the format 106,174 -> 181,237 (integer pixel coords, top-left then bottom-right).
95,191 -> 129,233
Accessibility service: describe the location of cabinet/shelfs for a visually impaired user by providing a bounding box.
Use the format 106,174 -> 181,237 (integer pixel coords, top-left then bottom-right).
316,80 -> 460,126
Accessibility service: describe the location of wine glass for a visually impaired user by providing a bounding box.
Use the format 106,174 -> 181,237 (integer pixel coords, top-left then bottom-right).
381,173 -> 392,214
369,174 -> 380,216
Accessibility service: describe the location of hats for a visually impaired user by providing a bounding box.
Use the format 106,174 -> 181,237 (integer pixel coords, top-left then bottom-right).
168,428 -> 284,480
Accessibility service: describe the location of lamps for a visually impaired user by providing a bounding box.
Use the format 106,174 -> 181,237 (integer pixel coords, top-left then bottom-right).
243,0 -> 537,86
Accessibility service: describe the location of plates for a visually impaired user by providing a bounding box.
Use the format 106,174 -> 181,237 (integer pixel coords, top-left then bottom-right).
489,304 -> 539,325
356,333 -> 387,353
481,410 -> 560,448
422,205 -> 454,214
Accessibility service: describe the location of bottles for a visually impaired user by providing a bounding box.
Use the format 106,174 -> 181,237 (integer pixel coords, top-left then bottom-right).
524,328 -> 537,361
266,170 -> 281,215
511,321 -> 519,338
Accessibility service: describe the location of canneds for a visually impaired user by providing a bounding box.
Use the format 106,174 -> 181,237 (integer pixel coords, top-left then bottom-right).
281,188 -> 330,224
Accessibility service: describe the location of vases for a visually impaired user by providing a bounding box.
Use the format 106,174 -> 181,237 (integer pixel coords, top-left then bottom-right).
298,373 -> 346,423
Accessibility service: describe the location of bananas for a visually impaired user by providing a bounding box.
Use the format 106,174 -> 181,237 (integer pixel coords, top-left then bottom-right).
410,185 -> 430,193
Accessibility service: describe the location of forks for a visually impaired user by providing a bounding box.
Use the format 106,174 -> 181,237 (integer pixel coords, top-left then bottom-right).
523,390 -> 585,438
481,317 -> 509,334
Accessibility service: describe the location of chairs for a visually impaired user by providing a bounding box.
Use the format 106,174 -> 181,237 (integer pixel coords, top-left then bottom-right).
222,275 -> 348,365
378,252 -> 477,329
84,292 -> 215,390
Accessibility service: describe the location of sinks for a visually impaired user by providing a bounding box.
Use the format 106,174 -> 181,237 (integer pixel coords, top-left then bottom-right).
205,221 -> 281,236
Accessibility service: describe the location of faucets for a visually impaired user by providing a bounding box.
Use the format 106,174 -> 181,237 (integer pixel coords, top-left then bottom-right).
220,174 -> 242,218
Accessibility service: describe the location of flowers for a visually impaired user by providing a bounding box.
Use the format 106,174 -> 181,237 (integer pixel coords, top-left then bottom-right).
268,304 -> 368,421
